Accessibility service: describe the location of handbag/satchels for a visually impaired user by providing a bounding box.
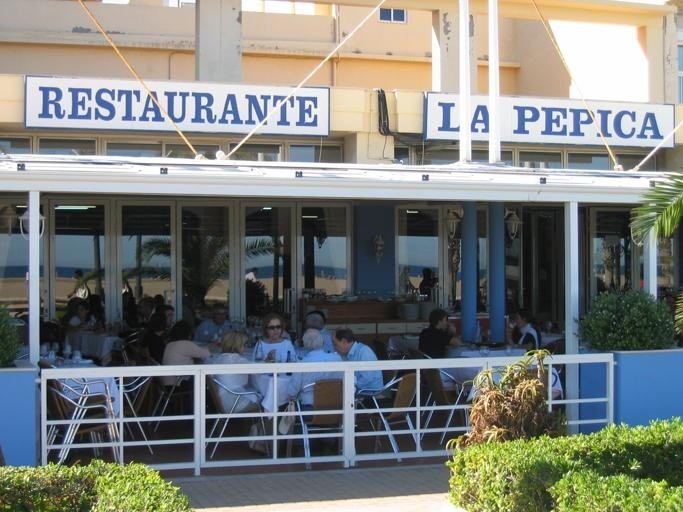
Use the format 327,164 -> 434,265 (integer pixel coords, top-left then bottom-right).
248,418 -> 280,454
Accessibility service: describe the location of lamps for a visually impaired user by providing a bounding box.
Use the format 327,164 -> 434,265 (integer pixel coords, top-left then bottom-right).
441,207 -> 464,240
504,207 -> 522,241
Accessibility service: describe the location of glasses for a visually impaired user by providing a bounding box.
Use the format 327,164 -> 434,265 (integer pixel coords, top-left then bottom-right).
266,325 -> 281,329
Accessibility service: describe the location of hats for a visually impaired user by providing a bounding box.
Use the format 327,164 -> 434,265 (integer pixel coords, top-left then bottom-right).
278,403 -> 295,434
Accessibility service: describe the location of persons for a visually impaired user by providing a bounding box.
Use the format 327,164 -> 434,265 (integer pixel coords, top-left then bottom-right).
418,307 -> 467,386
511,308 -> 542,356
332,326 -> 384,397
295,327 -> 358,449
246,267 -> 257,282
155,304 -> 174,329
61,291 -> 165,331
161,320 -> 212,392
204,329 -> 260,413
400,266 -> 438,301
294,314 -> 335,352
141,312 -> 166,360
194,303 -> 232,343
248,312 -> 300,398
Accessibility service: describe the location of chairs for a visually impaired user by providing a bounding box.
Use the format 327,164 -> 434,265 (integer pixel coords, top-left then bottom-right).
33,320 -> 567,473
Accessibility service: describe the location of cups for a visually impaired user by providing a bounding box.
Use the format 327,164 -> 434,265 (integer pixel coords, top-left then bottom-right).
40,342 -> 60,356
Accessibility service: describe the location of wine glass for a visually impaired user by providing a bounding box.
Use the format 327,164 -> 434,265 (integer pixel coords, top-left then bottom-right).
62,344 -> 82,368
